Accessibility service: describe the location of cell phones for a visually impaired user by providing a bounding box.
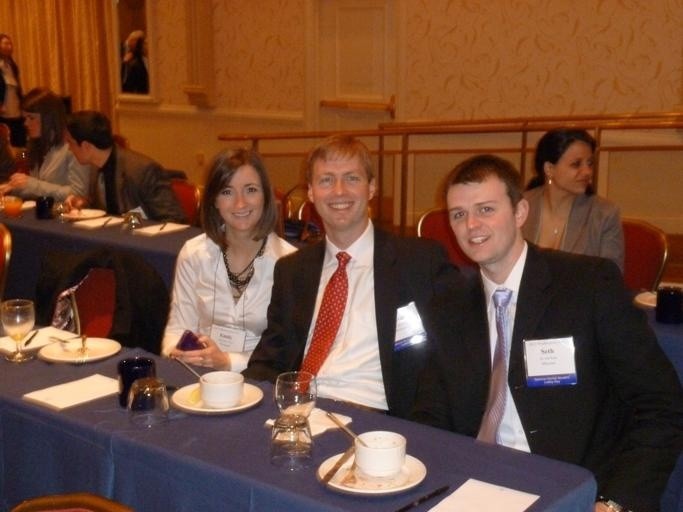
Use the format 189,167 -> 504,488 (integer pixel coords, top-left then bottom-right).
175,329 -> 204,351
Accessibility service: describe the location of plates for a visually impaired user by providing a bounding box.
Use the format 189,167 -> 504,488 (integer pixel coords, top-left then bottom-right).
635,291 -> 658,307
171,382 -> 264,416
318,452 -> 427,495
61,208 -> 106,220
0,200 -> 36,210
38,337 -> 122,363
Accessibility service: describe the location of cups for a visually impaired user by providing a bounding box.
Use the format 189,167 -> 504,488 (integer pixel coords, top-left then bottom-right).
117,357 -> 157,411
199,371 -> 245,409
354,432 -> 406,476
270,415 -> 318,471
36,196 -> 55,220
125,377 -> 170,427
655,286 -> 682,324
121,213 -> 143,238
2,195 -> 23,218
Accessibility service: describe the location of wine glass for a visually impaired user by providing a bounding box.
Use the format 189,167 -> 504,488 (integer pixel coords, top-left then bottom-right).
0,299 -> 35,362
274,371 -> 318,453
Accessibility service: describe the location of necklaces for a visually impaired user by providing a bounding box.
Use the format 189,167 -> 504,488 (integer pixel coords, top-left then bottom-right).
221,233 -> 268,287
546,198 -> 568,235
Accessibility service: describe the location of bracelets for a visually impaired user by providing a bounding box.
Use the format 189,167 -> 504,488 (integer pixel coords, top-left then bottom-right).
597,499 -> 623,512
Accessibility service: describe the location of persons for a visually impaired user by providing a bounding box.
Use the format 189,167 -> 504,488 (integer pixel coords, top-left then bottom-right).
0,88 -> 88,298
2,35 -> 25,148
160,146 -> 299,372
241,133 -> 449,426
408,154 -> 683,512
0,130 -> 16,177
518,127 -> 623,273
121,30 -> 148,92
57,110 -> 184,348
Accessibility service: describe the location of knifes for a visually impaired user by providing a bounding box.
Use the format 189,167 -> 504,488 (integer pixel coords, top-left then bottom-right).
318,446 -> 354,488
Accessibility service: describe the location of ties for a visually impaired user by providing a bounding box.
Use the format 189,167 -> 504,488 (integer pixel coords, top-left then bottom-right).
475,288 -> 514,441
96,171 -> 107,211
289,251 -> 353,395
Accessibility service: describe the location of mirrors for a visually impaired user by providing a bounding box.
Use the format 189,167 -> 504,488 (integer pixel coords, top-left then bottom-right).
112,0 -> 159,104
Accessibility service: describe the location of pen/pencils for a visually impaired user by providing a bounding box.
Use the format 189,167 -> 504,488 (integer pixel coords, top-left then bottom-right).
159,221 -> 168,231
397,485 -> 449,511
102,218 -> 112,228
